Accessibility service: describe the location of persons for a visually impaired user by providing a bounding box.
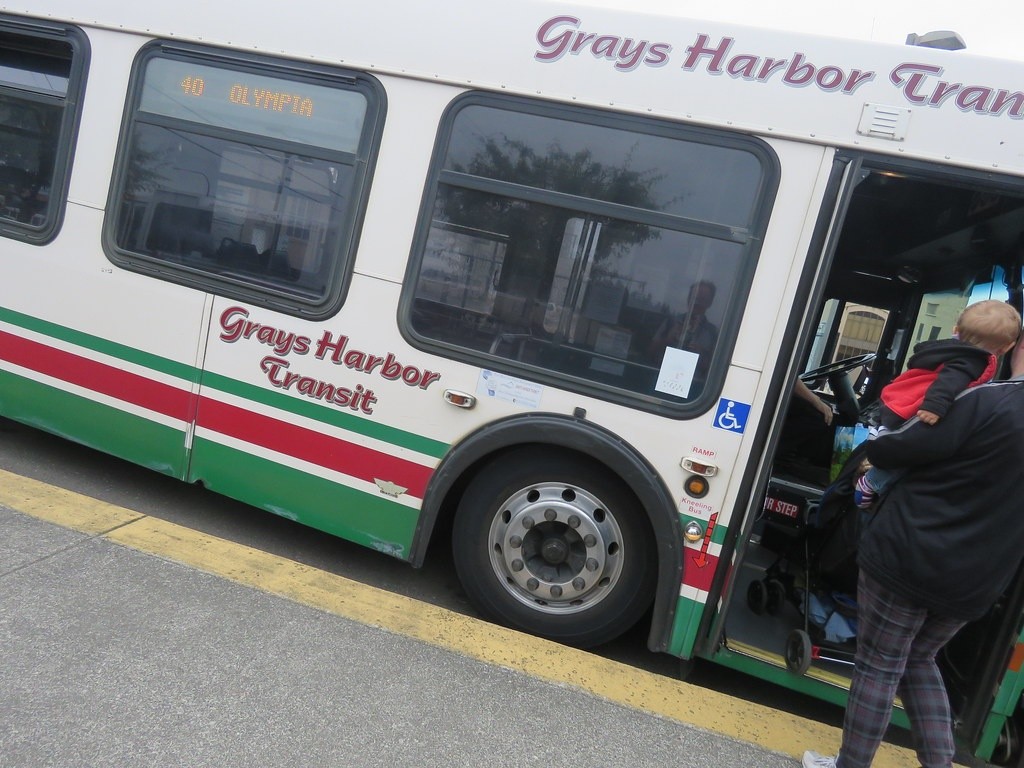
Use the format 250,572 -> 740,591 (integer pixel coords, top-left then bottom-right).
801,326 -> 1024,768
648,281 -> 720,383
775,376 -> 835,457
856,299 -> 1024,509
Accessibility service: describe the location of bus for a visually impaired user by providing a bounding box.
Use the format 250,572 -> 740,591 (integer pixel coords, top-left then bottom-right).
0,0 -> 1024,768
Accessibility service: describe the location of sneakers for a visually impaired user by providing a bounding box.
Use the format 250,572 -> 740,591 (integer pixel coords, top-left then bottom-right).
801,751 -> 839,768
